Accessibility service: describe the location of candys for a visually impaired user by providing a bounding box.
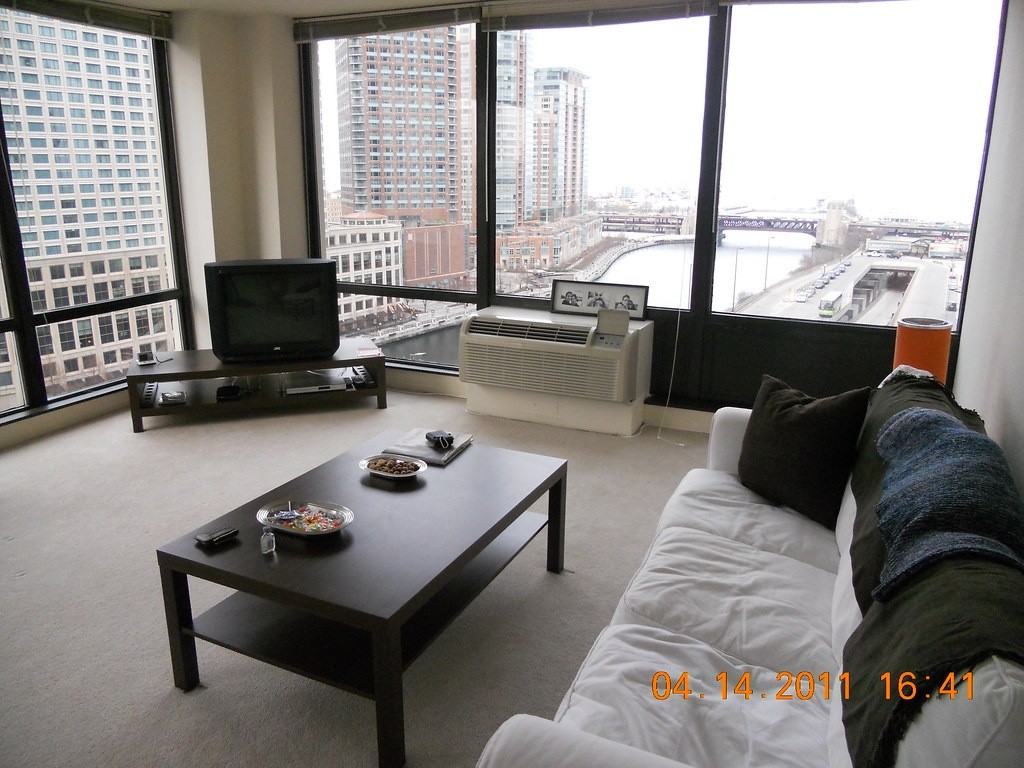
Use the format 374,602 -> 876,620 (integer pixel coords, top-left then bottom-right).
265,500 -> 343,532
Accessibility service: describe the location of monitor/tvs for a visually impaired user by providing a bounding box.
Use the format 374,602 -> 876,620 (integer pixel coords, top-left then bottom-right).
204,258 -> 340,365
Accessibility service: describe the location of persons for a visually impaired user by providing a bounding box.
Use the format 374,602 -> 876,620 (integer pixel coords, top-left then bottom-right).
616,295 -> 636,310
588,291 -> 608,308
562,291 -> 579,306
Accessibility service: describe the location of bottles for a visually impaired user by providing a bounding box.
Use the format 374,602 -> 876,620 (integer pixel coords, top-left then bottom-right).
261,526 -> 275,554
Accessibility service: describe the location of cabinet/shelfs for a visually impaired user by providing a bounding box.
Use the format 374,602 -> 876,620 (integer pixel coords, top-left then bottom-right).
126,337 -> 387,433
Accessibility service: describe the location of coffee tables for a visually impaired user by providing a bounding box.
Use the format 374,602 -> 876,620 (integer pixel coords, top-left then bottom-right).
156,433 -> 567,768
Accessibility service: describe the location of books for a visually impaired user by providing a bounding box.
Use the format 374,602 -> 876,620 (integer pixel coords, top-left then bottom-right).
382,427 -> 474,466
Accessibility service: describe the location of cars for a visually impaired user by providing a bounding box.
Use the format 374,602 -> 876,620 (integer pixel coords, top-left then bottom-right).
947,302 -> 956,312
865,250 -> 904,259
795,259 -> 852,303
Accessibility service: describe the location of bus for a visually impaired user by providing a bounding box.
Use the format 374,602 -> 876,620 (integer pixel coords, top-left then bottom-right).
818,293 -> 844,318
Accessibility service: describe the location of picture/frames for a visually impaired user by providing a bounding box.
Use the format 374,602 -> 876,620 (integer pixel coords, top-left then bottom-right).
550,279 -> 649,321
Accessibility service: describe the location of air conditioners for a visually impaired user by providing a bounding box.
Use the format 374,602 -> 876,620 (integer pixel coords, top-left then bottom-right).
459,304 -> 654,436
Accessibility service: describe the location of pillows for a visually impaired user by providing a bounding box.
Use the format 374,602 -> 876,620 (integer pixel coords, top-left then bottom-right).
736,372 -> 873,530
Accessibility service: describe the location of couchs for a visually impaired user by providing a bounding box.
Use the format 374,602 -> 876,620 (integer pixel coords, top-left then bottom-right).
477,366 -> 1024,768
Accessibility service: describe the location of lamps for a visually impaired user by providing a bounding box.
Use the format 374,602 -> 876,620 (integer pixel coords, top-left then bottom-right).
892,317 -> 954,385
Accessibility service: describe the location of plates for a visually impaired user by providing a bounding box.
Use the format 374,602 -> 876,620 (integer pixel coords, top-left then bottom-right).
256,499 -> 355,536
359,453 -> 427,479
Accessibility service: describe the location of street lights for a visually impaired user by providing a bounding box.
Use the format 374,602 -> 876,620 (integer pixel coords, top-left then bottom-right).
732,237 -> 777,311
